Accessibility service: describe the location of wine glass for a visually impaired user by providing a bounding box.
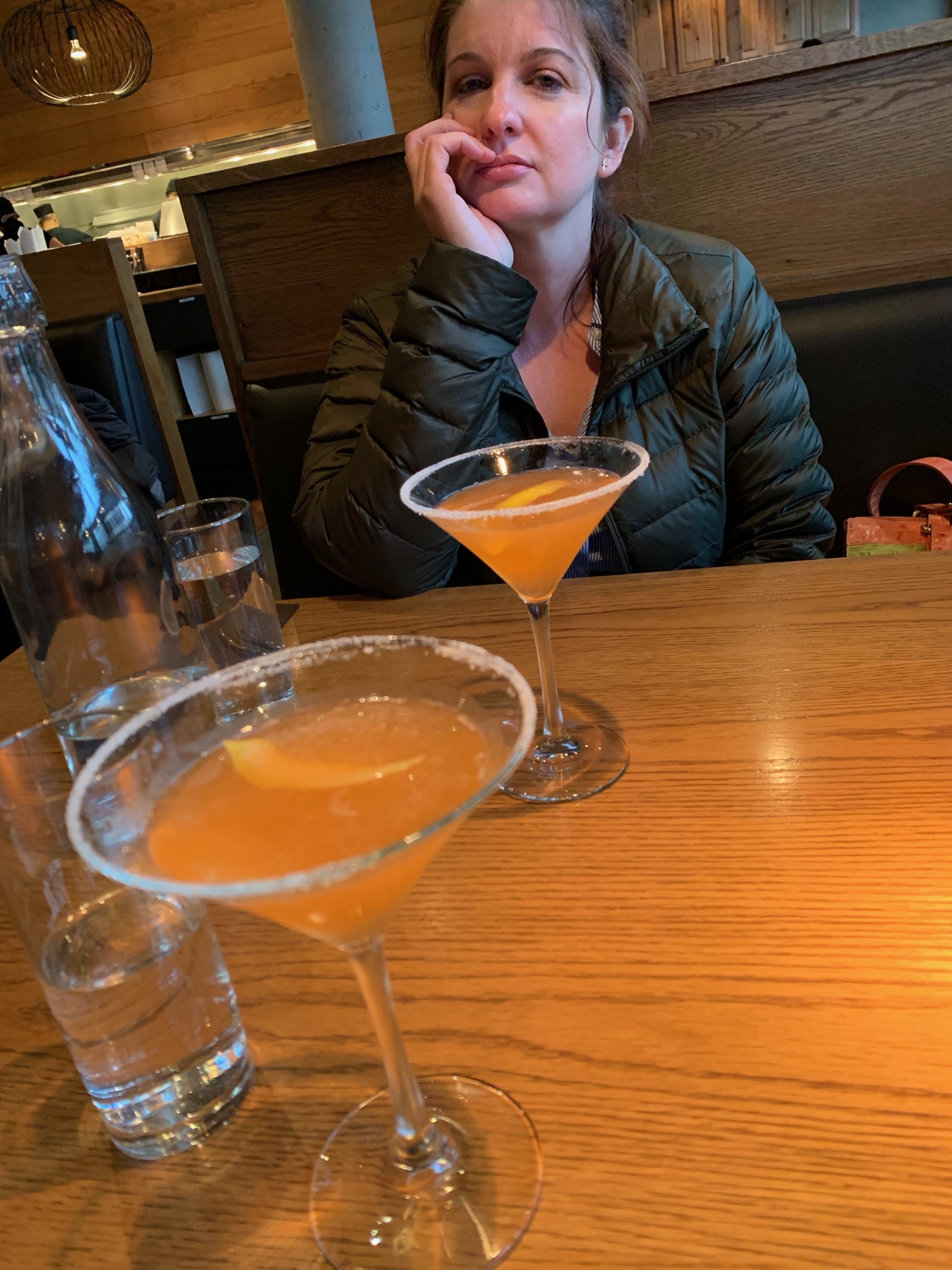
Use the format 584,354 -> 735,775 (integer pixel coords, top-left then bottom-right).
396,436 -> 653,802
65,629 -> 549,1269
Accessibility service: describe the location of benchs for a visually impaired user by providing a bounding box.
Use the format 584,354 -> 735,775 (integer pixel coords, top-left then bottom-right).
44,311 -> 189,533
247,279 -> 951,606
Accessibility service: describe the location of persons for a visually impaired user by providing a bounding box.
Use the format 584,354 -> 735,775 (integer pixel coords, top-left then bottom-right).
289,0 -> 837,600
33,203 -> 93,245
0,196 -> 66,256
152,178 -> 179,236
0,355 -> 170,661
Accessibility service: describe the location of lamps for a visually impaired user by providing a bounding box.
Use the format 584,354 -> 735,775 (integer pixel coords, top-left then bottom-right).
1,1 -> 153,108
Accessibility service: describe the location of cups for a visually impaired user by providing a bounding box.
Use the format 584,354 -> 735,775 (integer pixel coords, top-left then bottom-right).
0,703 -> 260,1162
155,496 -> 298,736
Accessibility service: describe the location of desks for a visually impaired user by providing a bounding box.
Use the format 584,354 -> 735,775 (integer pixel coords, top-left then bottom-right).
1,549 -> 951,1270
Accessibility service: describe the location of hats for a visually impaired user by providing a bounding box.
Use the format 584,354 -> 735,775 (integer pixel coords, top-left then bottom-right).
165,178 -> 180,196
33,203 -> 54,218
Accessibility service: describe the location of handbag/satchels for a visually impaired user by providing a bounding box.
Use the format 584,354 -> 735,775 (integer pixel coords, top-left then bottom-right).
842,457 -> 951,557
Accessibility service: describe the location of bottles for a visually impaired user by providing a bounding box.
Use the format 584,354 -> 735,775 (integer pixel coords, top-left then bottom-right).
1,255 -> 223,844
